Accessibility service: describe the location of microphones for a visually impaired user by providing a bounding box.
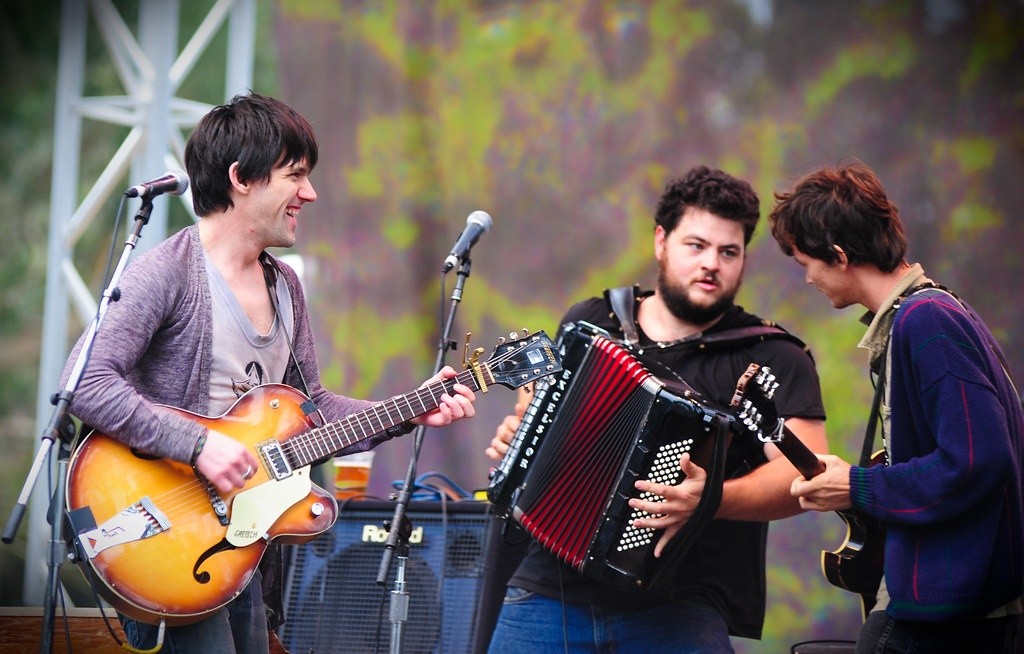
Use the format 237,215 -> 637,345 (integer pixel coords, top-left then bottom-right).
441,210 -> 493,274
123,168 -> 188,198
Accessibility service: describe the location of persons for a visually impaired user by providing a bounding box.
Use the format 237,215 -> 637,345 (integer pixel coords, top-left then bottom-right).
58,94 -> 475,654
769,163 -> 1024,654
486,164 -> 826,654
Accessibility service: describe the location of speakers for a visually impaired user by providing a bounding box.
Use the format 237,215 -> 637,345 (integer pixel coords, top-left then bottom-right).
271,499 -> 537,654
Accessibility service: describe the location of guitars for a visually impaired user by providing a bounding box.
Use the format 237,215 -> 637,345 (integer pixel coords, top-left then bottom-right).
65,327 -> 563,627
729,363 -> 889,626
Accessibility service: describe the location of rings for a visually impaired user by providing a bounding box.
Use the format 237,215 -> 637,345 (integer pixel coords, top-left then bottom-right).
242,465 -> 251,478
